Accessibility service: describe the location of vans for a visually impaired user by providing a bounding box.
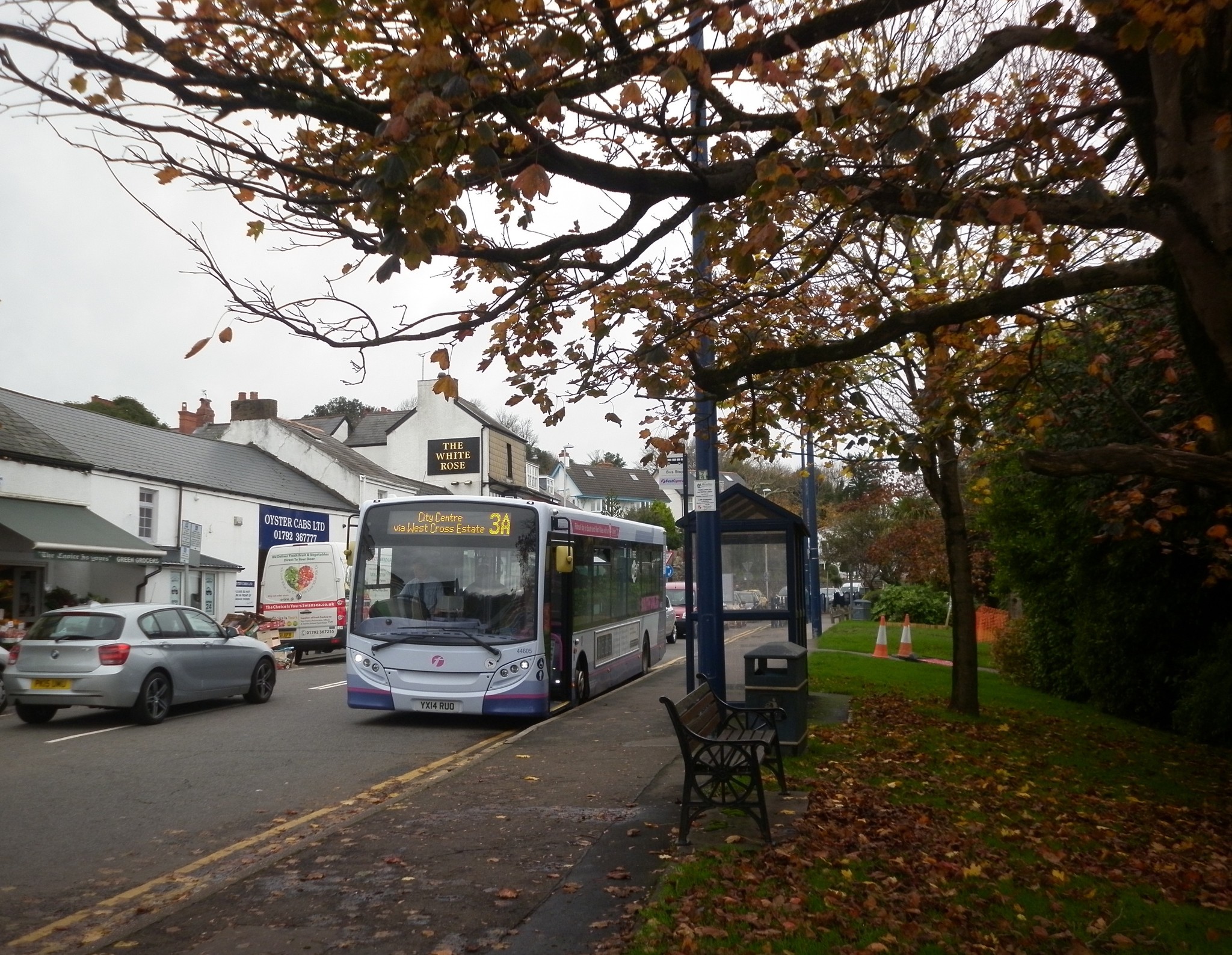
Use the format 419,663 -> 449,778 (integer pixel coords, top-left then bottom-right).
257,541 -> 403,664
666,581 -> 702,640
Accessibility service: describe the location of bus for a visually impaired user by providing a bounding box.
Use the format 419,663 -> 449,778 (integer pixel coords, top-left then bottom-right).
344,494 -> 668,718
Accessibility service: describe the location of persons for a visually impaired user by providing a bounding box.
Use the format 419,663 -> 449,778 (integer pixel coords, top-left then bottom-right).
463,564 -> 509,619
399,559 -> 441,616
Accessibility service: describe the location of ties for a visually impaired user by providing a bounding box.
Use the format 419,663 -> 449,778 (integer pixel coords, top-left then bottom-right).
419,582 -> 424,601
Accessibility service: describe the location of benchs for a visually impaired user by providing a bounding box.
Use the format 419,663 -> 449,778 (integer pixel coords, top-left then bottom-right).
656,674 -> 786,845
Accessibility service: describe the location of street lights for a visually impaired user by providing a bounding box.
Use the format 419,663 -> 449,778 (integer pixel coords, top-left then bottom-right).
563,445 -> 574,508
762,488 -> 772,607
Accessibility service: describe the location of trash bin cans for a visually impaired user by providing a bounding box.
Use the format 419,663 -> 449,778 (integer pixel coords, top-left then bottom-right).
744,642 -> 809,755
851,598 -> 872,621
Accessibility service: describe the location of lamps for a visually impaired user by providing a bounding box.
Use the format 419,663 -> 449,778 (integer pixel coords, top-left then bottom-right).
234,516 -> 242,525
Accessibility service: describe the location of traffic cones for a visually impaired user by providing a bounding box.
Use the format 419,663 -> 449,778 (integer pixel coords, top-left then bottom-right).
892,613 -> 921,659
872,615 -> 890,658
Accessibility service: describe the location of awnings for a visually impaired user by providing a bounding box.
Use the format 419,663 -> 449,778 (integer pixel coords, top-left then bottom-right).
0,496 -> 166,567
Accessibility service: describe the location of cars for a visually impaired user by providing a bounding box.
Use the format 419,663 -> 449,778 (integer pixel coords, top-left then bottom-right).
666,593 -> 678,645
775,585 -> 810,609
722,589 -> 770,632
1,601 -> 278,726
0,642 -> 17,715
839,582 -> 863,596
818,586 -> 844,603
